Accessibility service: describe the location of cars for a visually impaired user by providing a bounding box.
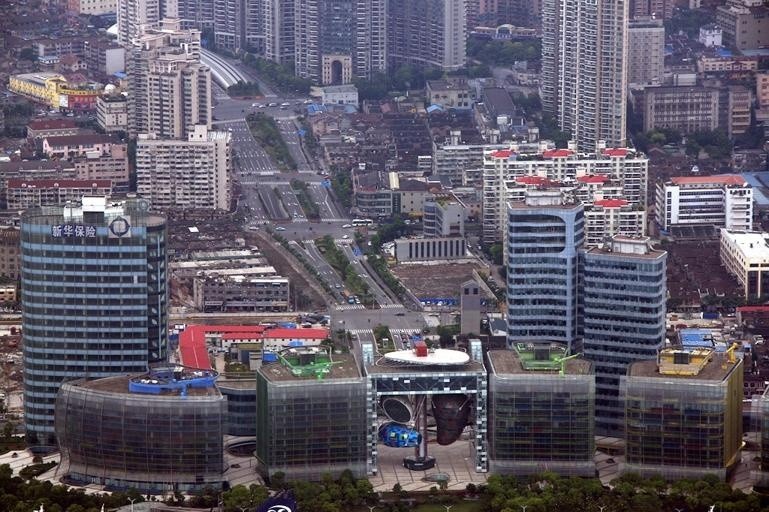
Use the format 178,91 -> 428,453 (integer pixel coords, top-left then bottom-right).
326,172 -> 328,176
282,190 -> 304,218
323,207 -> 327,212
336,283 -> 341,289
315,201 -> 325,206
428,311 -> 440,316
342,224 -> 351,228
34,108 -> 80,119
342,234 -> 349,239
320,171 -> 325,175
276,118 -> 291,125
394,312 -> 405,316
394,331 -> 422,350
338,289 -> 361,304
251,99 -> 313,110
467,242 -> 481,251
275,227 -> 285,231
313,185 -> 316,189
250,226 -> 259,230
450,311 -> 460,315
1,408 -> 23,420
342,283 -> 344,288
0,306 -> 22,314
231,463 -> 241,469
228,126 -> 233,132
403,219 -> 419,226
251,207 -> 265,220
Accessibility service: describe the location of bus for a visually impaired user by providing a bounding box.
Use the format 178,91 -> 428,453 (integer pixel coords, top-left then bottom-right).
352,219 -> 373,226
